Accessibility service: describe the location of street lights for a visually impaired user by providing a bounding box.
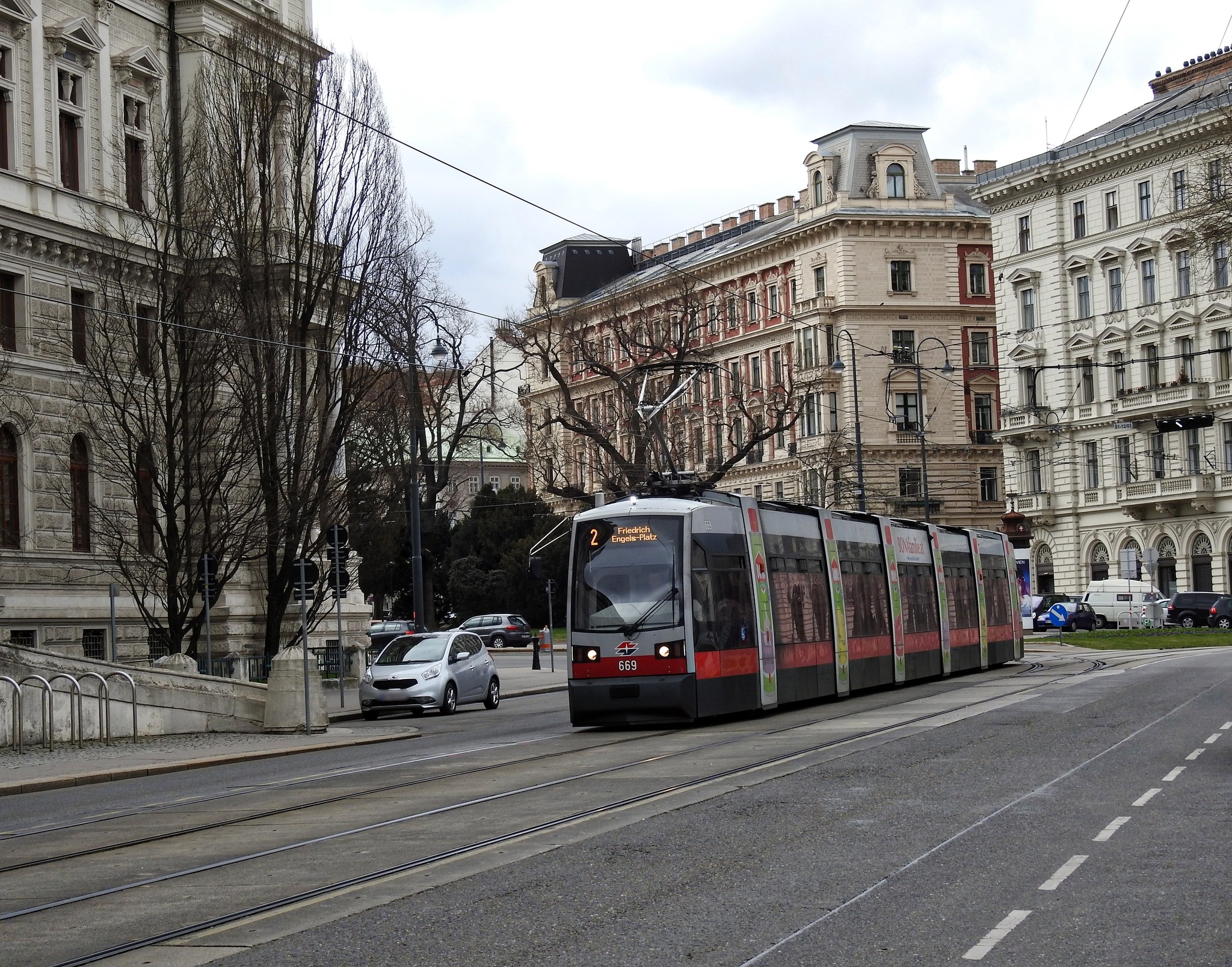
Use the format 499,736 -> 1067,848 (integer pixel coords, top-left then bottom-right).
479,423 -> 507,490
404,306 -> 449,621
912,337 -> 953,524
830,329 -> 866,513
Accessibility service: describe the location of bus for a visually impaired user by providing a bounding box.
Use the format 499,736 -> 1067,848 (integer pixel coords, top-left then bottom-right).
527,471 -> 1022,729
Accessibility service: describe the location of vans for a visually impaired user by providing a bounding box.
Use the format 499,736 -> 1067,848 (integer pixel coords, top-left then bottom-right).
1079,579 -> 1168,628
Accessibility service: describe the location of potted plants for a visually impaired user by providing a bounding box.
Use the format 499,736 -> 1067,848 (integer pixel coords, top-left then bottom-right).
1177,365 -> 1190,385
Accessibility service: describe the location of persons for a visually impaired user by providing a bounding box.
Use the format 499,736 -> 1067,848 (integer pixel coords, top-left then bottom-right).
787,582 -> 827,642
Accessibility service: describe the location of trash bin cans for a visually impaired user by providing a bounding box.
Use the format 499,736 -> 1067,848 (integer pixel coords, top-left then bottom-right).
539,627 -> 551,652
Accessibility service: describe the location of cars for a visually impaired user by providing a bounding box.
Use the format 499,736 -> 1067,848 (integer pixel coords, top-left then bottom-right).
1141,597 -> 1173,628
443,614 -> 532,647
1208,598 -> 1232,628
358,632 -> 503,716
1037,602 -> 1096,631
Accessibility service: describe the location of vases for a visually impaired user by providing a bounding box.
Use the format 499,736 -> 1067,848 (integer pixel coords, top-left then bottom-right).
1120,382 -> 1179,395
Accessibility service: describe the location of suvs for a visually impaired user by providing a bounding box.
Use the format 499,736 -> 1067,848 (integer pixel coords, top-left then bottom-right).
369,620 -> 430,652
1167,592 -> 1232,629
1033,592 -> 1071,632
